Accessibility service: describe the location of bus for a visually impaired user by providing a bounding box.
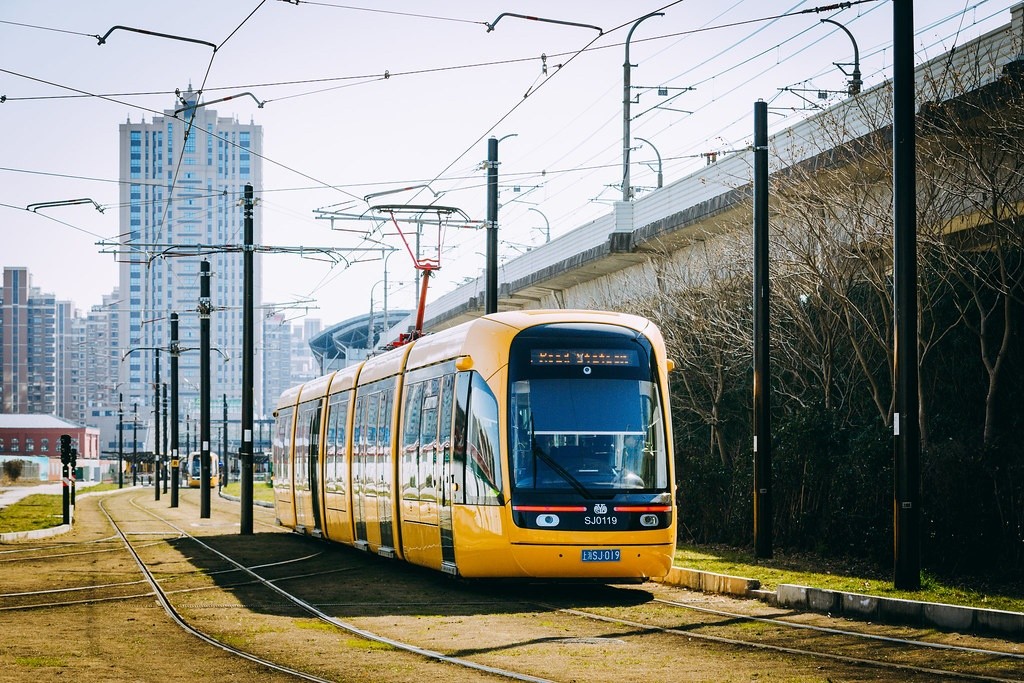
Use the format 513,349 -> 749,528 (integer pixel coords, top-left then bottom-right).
186,449 -> 220,489
271,205 -> 679,583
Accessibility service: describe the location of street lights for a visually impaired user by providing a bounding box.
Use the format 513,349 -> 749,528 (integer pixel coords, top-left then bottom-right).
619,12 -> 666,203
633,136 -> 663,188
819,17 -> 861,94
384,247 -> 400,333
528,208 -> 550,242
